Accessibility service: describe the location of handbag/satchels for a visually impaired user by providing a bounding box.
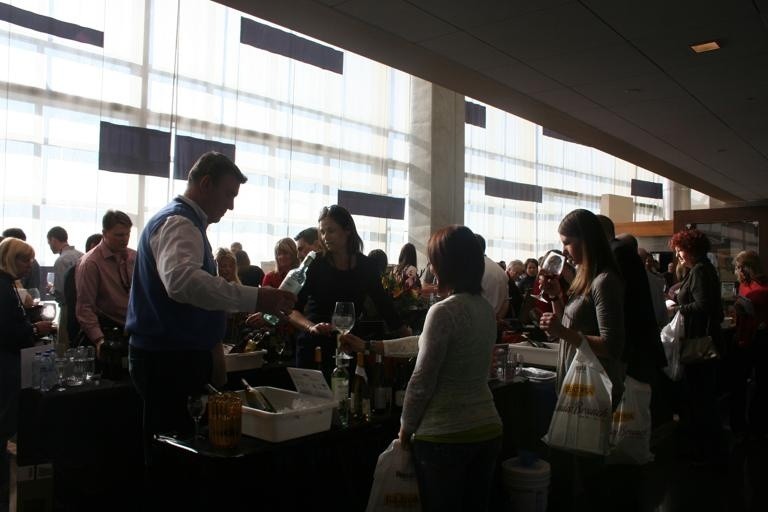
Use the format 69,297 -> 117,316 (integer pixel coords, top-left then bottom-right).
609,377 -> 658,470
544,334 -> 615,459
365,437 -> 422,512
661,298 -> 721,383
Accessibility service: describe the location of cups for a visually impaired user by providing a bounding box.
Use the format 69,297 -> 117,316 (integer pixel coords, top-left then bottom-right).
66,347 -> 95,386
209,396 -> 241,447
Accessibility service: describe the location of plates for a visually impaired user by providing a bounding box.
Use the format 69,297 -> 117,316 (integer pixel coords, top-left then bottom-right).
524,366 -> 557,382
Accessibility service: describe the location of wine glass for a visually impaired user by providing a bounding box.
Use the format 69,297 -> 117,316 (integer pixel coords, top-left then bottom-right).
56,358 -> 67,391
529,252 -> 566,305
187,394 -> 207,438
40,303 -> 56,340
333,302 -> 353,360
46,274 -> 54,296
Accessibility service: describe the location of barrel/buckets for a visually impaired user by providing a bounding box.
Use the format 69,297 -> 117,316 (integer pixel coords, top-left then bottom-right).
504,458 -> 555,510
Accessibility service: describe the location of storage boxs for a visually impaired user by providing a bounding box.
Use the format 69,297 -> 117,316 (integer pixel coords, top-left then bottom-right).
231,386 -> 340,443
509,342 -> 559,367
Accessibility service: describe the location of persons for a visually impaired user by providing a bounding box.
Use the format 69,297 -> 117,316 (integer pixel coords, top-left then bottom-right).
0,151 -> 768,510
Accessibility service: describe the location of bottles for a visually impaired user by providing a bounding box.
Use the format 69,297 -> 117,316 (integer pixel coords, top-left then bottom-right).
32,349 -> 56,392
101,326 -> 128,381
329,352 -> 407,429
521,332 -> 551,351
206,382 -> 241,398
240,377 -> 276,413
229,251 -> 316,354
